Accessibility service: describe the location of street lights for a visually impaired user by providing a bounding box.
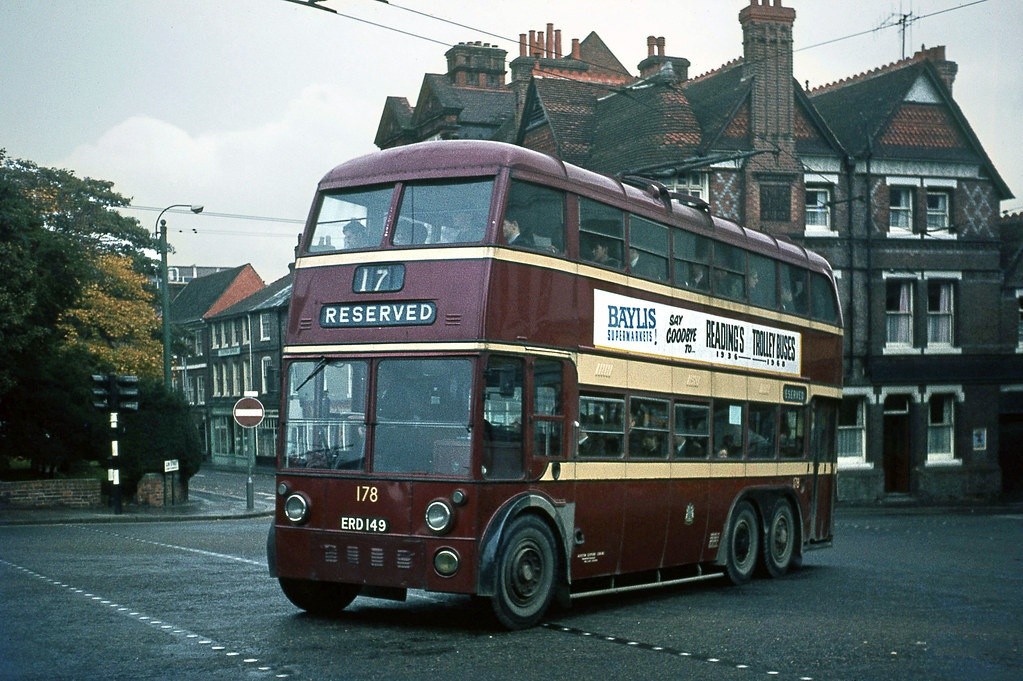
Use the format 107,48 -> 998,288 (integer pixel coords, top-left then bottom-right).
153,204 -> 204,397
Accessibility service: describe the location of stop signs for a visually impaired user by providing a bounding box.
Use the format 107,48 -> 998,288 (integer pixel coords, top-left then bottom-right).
232,396 -> 266,429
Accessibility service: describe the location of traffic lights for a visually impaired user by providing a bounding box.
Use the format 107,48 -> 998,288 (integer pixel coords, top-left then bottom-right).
116,374 -> 139,413
90,373 -> 110,409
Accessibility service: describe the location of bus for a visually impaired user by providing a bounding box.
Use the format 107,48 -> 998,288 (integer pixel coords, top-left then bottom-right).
263,139 -> 845,630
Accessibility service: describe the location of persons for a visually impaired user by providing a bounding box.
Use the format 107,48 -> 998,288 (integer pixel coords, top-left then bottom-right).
357,363 -> 411,454
592,238 -> 618,268
578,405 -> 791,458
393,201 -> 534,247
629,239 -> 803,314
343,218 -> 371,248
